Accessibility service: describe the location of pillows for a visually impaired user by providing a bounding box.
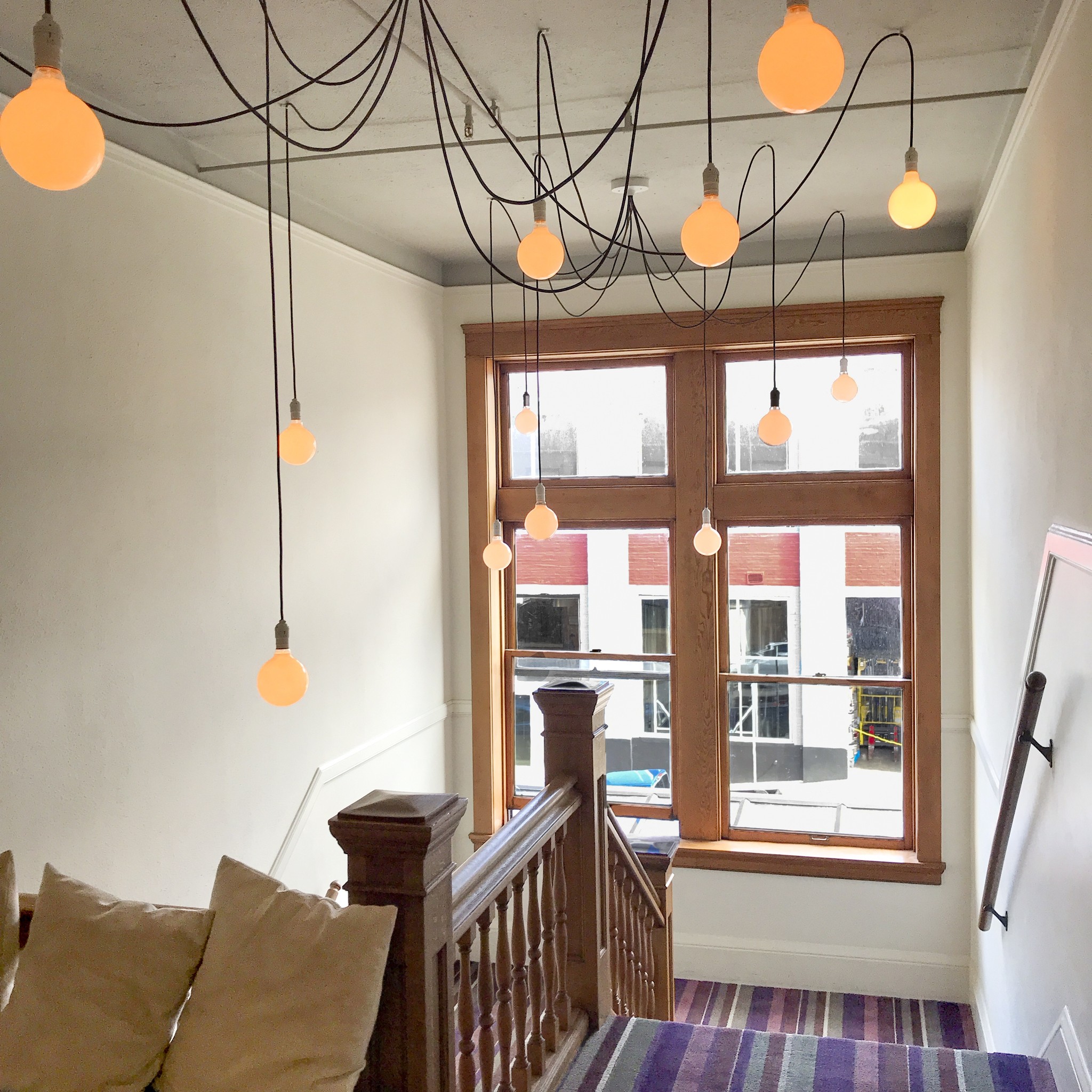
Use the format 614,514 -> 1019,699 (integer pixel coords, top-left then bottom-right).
153,854 -> 399,1091
0,862 -> 214,1092
